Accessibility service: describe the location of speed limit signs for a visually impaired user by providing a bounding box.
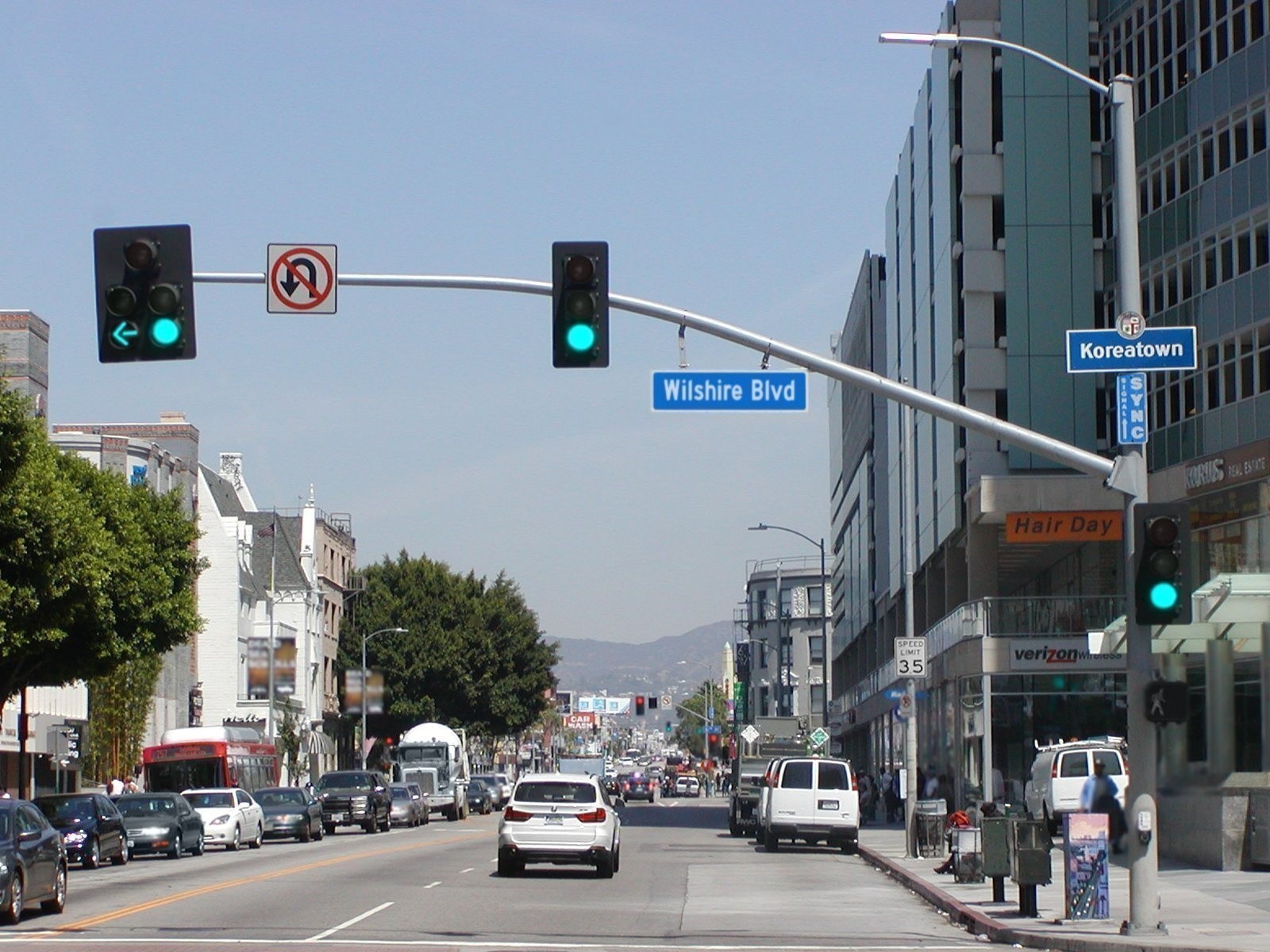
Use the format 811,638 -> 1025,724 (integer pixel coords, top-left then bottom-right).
895,637 -> 927,677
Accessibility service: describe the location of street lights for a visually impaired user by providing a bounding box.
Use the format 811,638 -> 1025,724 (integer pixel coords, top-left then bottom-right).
747,523 -> 829,727
677,659 -> 713,759
737,639 -> 782,718
739,600 -> 790,717
551,704 -> 572,772
269,590 -> 328,744
361,628 -> 410,772
878,30 -> 1169,938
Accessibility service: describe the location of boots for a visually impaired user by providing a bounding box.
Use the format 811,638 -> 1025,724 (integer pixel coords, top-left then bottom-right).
934,858 -> 953,876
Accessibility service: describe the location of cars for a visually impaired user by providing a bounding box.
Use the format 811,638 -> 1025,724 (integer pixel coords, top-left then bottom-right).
0,798 -> 67,925
557,746 -> 731,804
170,788 -> 265,852
408,781 -> 430,825
466,779 -> 493,815
115,792 -> 204,860
30,793 -> 128,869
251,786 -> 323,843
388,784 -> 421,828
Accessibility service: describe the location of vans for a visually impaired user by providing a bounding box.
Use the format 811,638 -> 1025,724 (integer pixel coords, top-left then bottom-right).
1024,736 -> 1129,836
759,756 -> 860,855
751,756 -> 781,846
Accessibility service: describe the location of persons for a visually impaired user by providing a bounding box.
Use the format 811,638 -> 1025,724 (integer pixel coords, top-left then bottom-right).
857,768 -> 940,827
1080,755 -> 1123,853
655,766 -> 730,797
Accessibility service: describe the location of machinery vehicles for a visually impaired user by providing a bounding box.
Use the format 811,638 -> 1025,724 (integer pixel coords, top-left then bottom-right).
390,722 -> 469,821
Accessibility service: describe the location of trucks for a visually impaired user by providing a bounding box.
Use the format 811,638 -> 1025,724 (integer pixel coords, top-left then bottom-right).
729,716 -> 808,837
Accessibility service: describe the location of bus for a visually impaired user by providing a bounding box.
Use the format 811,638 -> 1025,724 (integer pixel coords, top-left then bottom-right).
135,726 -> 279,809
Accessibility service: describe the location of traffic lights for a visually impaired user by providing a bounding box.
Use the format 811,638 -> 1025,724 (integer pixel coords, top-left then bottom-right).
1143,680 -> 1186,723
1134,502 -> 1192,625
93,224 -> 198,363
636,696 -> 644,715
552,241 -> 609,369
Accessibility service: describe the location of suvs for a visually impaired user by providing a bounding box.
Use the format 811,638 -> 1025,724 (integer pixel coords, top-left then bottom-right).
305,770 -> 391,835
497,770 -> 621,878
470,775 -> 503,811
495,773 -> 515,807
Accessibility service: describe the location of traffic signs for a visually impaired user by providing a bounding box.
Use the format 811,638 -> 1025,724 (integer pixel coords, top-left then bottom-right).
709,707 -> 714,718
51,724 -> 82,770
651,370 -> 808,412
703,725 -> 721,733
1117,372 -> 1148,444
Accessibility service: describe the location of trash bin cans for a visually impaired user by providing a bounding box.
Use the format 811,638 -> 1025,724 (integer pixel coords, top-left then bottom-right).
952,827 -> 982,883
915,798 -> 945,857
